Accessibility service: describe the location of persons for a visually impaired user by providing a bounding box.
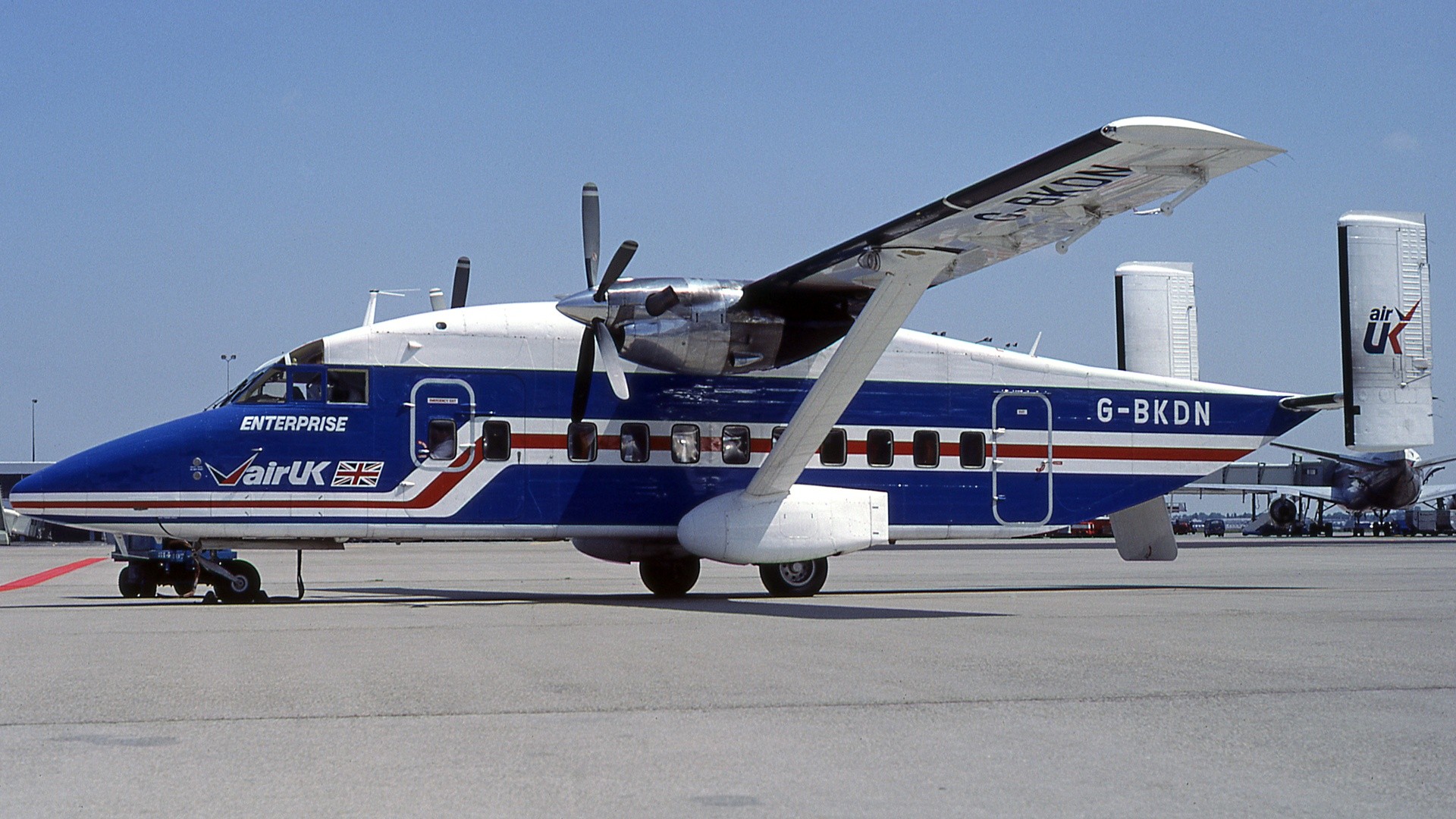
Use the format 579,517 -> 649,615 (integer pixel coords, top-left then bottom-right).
430,424 -> 455,458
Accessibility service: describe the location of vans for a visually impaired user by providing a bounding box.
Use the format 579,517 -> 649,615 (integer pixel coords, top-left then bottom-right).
1204,518 -> 1225,537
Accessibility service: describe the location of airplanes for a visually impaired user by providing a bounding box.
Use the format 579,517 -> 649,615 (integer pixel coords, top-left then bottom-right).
1181,442 -> 1456,538
10,113 -> 1432,603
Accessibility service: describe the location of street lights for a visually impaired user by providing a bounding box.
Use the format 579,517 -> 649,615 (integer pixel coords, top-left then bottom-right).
32,399 -> 37,462
221,355 -> 236,397
